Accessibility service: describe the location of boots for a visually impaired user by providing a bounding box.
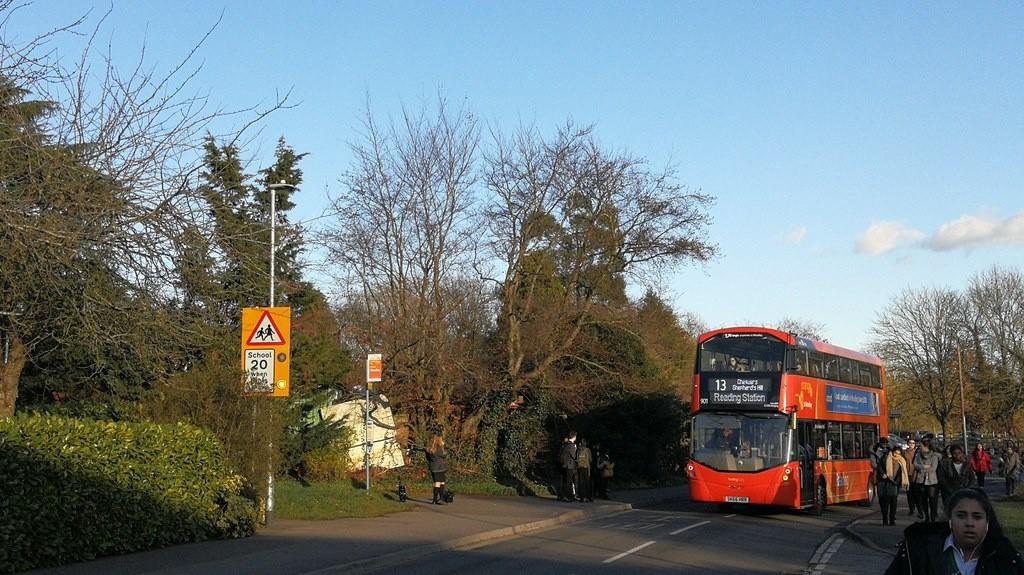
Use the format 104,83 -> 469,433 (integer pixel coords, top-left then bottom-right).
890,514 -> 896,526
922,505 -> 930,522
883,514 -> 888,526
432,484 -> 448,505
931,511 -> 937,521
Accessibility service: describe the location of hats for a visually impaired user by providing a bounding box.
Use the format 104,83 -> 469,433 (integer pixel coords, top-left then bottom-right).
879,437 -> 889,443
723,428 -> 733,436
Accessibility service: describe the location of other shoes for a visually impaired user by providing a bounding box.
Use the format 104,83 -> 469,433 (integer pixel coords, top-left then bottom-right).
579,497 -> 585,503
907,511 -> 914,515
560,496 -> 575,502
589,498 -> 594,503
918,512 -> 923,517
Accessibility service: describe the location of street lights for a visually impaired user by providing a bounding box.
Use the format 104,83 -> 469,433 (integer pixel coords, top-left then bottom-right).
264,182 -> 302,525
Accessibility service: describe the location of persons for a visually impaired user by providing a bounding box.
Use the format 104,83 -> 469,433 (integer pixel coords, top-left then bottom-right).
559,431 -> 614,503
999,446 -> 1020,495
727,357 -> 744,371
425,435 -> 448,505
884,486 -> 1024,575
870,437 -> 993,526
714,428 -> 736,451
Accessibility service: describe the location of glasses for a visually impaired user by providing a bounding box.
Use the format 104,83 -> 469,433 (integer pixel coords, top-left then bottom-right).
729,360 -> 735,363
908,441 -> 915,445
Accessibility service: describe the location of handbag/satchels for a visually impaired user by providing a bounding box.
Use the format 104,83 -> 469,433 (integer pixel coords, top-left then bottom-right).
911,469 -> 917,483
878,476 -> 897,498
444,488 -> 455,503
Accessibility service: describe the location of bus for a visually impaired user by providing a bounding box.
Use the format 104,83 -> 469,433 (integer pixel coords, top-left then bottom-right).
686,326 -> 889,514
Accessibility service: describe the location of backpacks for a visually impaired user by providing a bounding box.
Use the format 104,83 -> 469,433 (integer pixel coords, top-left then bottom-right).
576,447 -> 590,469
559,442 -> 572,468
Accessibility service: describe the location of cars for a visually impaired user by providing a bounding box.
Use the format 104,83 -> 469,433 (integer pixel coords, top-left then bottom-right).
915,430 -> 928,439
936,431 -> 953,442
888,432 -> 909,451
959,431 -> 971,440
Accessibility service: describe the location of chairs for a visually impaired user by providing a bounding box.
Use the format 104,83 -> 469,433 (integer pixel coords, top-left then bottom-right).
706,355 -> 817,377
